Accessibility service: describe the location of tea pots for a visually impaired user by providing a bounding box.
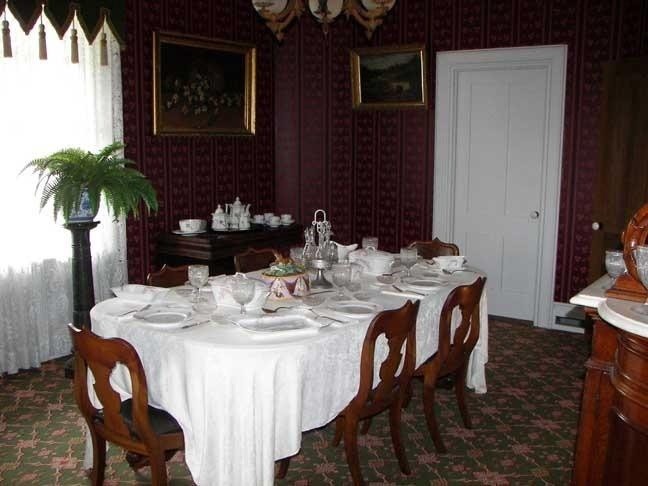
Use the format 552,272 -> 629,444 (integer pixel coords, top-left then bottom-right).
211,196 -> 252,230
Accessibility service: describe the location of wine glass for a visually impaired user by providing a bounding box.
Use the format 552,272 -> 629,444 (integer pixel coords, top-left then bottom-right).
400,248 -> 418,279
331,267 -> 353,302
229,278 -> 255,316
187,265 -> 211,303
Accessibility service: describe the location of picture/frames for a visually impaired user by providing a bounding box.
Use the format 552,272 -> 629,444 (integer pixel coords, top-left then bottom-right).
346,41 -> 431,112
151,29 -> 256,138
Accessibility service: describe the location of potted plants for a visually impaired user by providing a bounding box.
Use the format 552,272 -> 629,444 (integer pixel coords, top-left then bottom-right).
20,140 -> 162,225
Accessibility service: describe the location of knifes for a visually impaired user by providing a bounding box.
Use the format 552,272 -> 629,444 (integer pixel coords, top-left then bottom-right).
180,320 -> 209,330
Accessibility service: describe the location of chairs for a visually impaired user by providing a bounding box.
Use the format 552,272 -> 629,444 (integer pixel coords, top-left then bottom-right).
360,273 -> 487,458
403,236 -> 468,260
143,261 -> 208,287
235,245 -> 289,278
275,298 -> 422,484
60,318 -> 185,486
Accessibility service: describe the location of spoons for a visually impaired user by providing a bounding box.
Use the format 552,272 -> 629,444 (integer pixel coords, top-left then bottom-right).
261,305 -> 291,313
293,289 -> 336,299
383,270 -> 400,277
442,268 -> 470,275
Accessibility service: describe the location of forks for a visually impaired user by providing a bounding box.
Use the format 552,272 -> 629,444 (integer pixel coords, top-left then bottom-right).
392,285 -> 429,298
309,309 -> 344,323
119,303 -> 153,317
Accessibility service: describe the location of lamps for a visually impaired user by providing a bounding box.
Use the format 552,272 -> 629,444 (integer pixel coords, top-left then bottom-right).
247,0 -> 399,42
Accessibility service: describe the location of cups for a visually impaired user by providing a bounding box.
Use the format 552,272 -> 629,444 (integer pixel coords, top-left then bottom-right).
362,237 -> 380,256
253,212 -> 295,228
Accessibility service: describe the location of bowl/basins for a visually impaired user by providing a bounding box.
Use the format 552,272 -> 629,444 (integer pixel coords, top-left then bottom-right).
634,245 -> 648,290
432,255 -> 466,273
604,250 -> 625,277
335,242 -> 358,264
177,219 -> 202,233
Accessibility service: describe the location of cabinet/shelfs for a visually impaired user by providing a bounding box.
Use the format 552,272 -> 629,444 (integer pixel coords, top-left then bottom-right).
163,222 -> 306,276
566,271 -> 648,486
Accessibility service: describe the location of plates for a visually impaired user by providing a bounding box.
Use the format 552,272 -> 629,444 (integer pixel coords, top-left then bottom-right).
328,300 -> 384,320
407,279 -> 442,291
239,315 -> 316,333
114,284 -> 168,302
135,308 -> 193,327
171,230 -> 206,237
